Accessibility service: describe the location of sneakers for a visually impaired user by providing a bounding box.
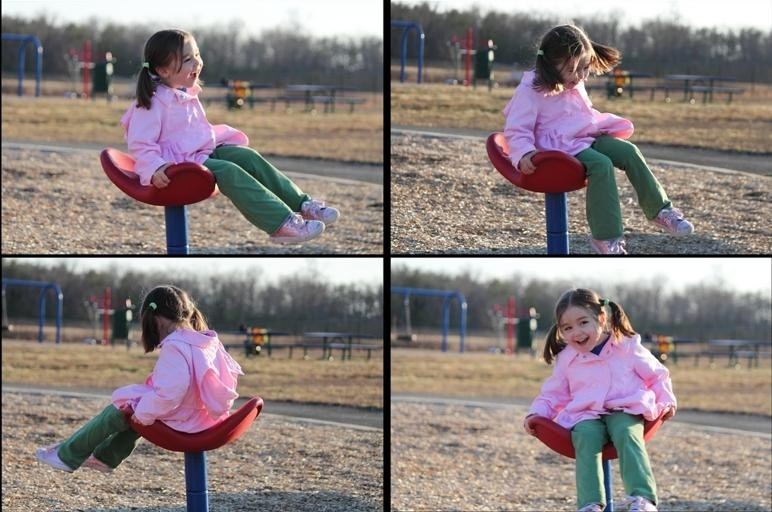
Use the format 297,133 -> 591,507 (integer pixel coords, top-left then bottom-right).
270,200 -> 340,243
590,237 -> 629,254
648,208 -> 694,236
579,503 -> 602,512
81,453 -> 116,473
629,495 -> 658,512
36,447 -> 74,473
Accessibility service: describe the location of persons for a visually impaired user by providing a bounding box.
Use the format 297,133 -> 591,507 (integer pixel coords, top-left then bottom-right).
524,288 -> 678,512
36,285 -> 242,473
121,30 -> 340,243
504,25 -> 694,255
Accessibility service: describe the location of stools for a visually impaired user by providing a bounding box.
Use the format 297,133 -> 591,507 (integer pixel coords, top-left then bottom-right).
120,397 -> 265,512
100,148 -> 215,255
487,133 -> 588,254
526,407 -> 666,511
